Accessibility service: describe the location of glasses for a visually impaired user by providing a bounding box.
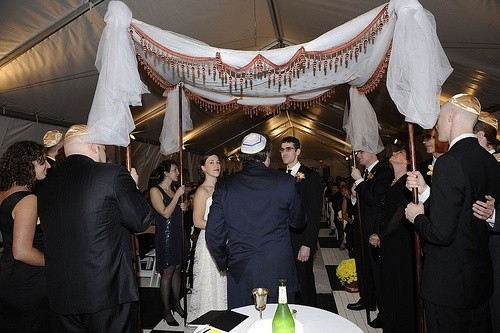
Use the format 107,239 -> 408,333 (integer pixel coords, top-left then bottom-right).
355,150 -> 364,155
422,135 -> 436,139
32,156 -> 47,165
279,147 -> 296,152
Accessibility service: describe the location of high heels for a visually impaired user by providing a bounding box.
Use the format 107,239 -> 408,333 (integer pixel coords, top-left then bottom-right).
162,311 -> 179,326
171,306 -> 185,318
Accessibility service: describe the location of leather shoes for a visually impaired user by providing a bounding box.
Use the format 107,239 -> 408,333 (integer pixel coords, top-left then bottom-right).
347,299 -> 377,311
370,313 -> 382,328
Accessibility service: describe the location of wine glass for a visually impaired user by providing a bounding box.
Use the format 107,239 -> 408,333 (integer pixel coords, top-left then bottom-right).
252,287 -> 269,319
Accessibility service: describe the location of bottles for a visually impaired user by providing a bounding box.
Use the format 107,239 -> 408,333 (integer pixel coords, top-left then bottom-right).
272,279 -> 295,333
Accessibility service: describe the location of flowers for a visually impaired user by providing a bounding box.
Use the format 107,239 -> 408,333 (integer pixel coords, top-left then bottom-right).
335,258 -> 360,284
295,171 -> 307,184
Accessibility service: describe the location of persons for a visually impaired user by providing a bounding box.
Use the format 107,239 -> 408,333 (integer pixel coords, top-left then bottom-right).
138,181 -> 199,295
37,124 -> 153,333
32,130 -> 64,250
278,136 -> 322,307
186,153 -> 228,322
323,126 -> 500,333
0,141 -> 52,333
205,132 -> 308,309
404,93 -> 500,333
149,161 -> 187,327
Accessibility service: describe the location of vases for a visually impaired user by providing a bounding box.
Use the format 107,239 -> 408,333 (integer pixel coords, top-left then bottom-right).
343,280 -> 360,293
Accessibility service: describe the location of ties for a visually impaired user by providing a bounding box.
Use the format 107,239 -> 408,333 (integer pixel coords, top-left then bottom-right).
364,169 -> 369,181
288,170 -> 292,174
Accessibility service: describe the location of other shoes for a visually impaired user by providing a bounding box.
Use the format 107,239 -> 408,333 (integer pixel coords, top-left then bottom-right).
329,230 -> 335,235
340,243 -> 346,249
336,240 -> 342,246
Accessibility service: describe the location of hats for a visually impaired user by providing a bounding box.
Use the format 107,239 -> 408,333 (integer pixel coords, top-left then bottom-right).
43,129 -> 63,148
241,133 -> 267,155
64,124 -> 88,140
478,112 -> 498,130
449,94 -> 481,115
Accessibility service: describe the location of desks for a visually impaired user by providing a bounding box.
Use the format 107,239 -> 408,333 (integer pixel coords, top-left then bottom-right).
184,303 -> 366,333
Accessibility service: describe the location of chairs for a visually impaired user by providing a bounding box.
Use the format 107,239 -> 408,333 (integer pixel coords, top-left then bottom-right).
322,186 -> 354,244
132,230 -> 162,289
